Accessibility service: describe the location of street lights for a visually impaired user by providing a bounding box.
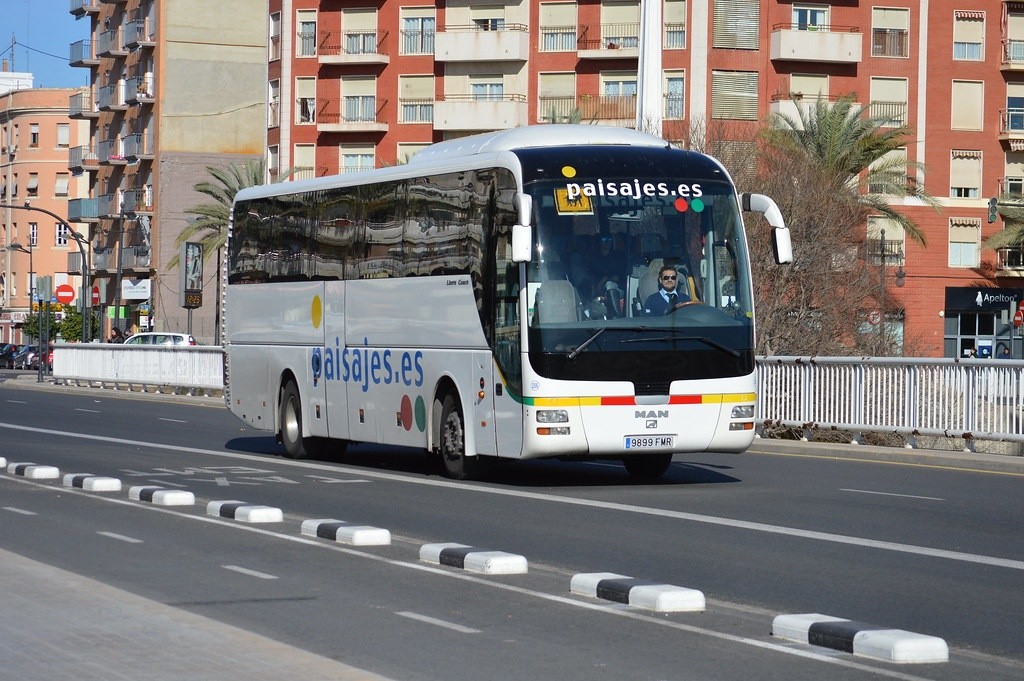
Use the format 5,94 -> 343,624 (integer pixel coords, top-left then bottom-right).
6,235 -> 34,345
114,201 -> 149,330
876,228 -> 907,353
62,231 -> 92,342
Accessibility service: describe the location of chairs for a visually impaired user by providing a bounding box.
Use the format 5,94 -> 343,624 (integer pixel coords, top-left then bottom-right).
637,268 -> 690,310
537,279 -> 582,323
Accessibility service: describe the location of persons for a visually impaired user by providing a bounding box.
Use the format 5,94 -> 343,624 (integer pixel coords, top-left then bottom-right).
997,348 -> 1010,359
540,225 -> 627,320
128,331 -> 132,336
970,348 -> 978,358
107,327 -> 125,344
188,246 -> 201,289
642,266 -> 692,317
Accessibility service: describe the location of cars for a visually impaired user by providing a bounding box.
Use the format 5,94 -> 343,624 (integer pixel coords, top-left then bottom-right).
122,332 -> 196,346
0,343 -> 53,371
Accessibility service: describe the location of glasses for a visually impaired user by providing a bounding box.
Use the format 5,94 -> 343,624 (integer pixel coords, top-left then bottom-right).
662,276 -> 677,280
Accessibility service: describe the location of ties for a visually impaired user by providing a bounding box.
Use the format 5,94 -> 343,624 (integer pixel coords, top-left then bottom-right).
666,293 -> 675,307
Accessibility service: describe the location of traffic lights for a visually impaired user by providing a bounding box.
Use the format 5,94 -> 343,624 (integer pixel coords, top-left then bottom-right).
988,196 -> 999,223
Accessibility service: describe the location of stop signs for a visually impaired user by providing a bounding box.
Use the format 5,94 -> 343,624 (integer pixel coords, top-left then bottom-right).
56,285 -> 74,304
91,285 -> 99,305
1018,300 -> 1024,316
1013,310 -> 1022,328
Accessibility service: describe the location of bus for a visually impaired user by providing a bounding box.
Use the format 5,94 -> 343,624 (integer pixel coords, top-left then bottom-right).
219,123 -> 794,481
